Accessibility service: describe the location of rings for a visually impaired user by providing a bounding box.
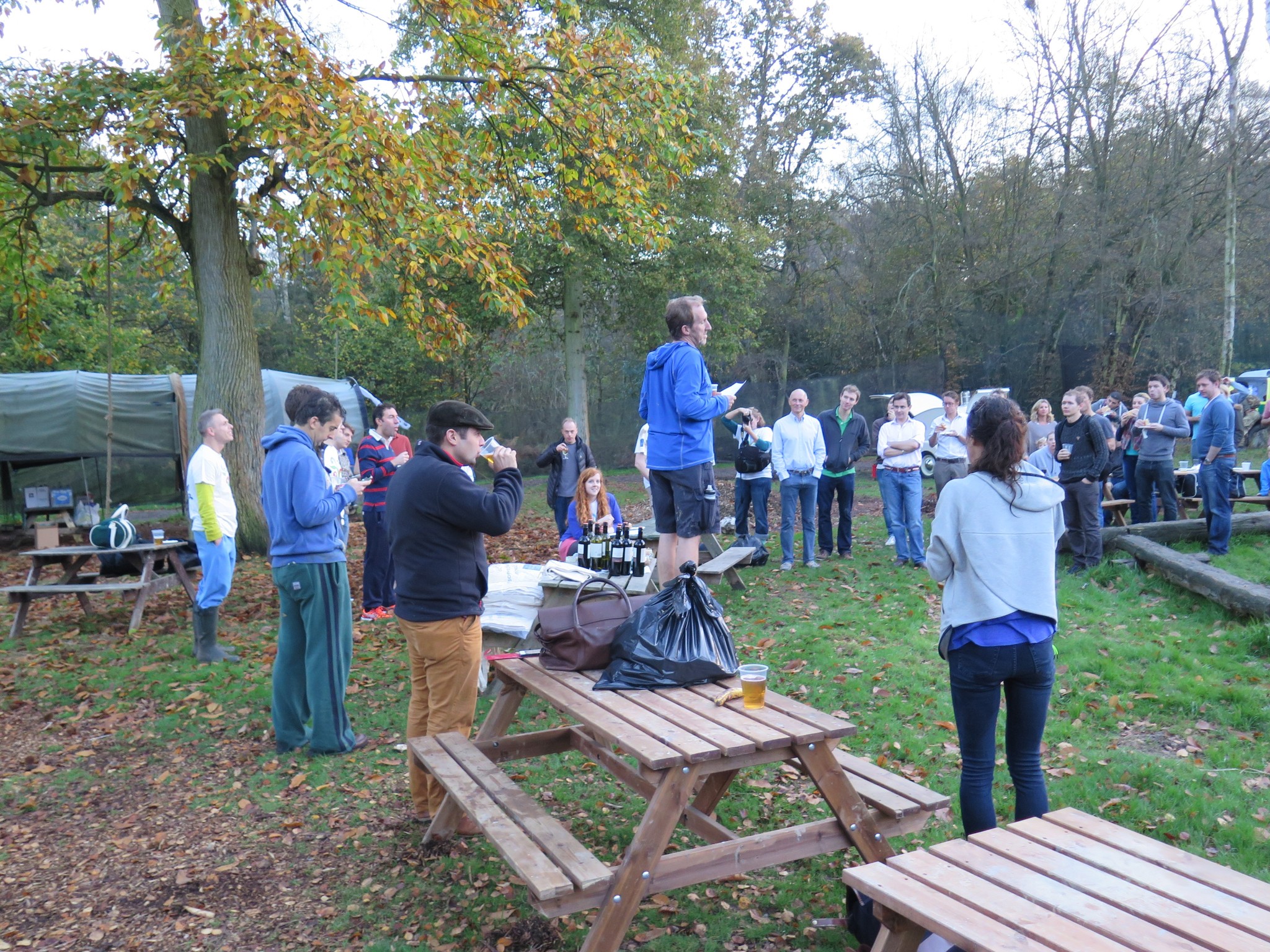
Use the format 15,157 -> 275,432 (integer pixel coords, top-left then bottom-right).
745,411 -> 747,414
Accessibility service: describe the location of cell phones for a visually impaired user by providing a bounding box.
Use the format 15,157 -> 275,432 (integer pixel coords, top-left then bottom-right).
361,474 -> 373,481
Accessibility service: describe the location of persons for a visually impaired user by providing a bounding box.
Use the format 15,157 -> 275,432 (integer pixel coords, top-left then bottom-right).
536,416 -> 599,539
638,296 -> 738,598
921,396 -> 1068,835
817,384 -> 871,562
184,408 -> 243,663
386,401 -> 523,837
771,388 -> 826,570
558,467 -> 624,567
283,384 -> 479,623
871,367 -> 1270,576
634,422 -> 654,513
720,406 -> 773,545
259,390 -> 374,759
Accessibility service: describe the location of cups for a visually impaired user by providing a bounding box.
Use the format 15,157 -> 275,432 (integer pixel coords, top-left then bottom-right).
737,664 -> 769,708
1242,462 -> 1251,471
151,529 -> 165,545
1062,443 -> 1074,460
711,384 -> 718,396
1179,461 -> 1188,472
479,437 -> 501,465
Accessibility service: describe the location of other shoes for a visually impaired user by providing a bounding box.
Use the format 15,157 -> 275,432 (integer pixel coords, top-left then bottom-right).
894,558 -> 909,567
1067,565 -> 1083,575
915,561 -> 928,569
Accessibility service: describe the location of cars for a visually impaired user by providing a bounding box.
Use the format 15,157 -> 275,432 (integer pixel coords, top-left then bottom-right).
868,386 -> 1010,478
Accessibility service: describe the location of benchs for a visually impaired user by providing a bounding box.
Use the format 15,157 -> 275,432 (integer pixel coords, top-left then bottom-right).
406,643 -> 948,952
694,544 -> 757,586
3,524 -> 21,547
3,538 -> 205,639
1098,465 -> 1270,525
78,524 -> 101,533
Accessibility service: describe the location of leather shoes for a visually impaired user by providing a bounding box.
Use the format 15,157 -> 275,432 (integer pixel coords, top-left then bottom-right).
453,815 -> 485,837
351,733 -> 368,750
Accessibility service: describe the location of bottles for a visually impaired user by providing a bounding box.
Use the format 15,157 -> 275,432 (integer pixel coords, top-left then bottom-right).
577,518 -> 645,577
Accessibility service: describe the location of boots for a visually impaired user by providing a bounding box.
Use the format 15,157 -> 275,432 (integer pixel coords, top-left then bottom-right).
194,607 -> 240,664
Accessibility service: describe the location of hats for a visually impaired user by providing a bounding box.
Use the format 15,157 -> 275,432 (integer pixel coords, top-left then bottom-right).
426,399 -> 496,431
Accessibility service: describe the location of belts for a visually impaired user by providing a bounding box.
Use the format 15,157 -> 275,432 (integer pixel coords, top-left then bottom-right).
936,457 -> 966,464
786,468 -> 813,474
883,466 -> 919,473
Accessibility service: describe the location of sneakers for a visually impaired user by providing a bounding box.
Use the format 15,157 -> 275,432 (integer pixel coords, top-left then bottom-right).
804,561 -> 820,568
885,536 -> 896,546
361,606 -> 393,620
840,553 -> 855,561
780,562 -> 792,571
816,549 -> 831,561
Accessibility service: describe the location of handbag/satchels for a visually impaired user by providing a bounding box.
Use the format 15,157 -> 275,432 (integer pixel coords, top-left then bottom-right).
592,561 -> 741,690
1228,472 -> 1247,498
727,535 -> 769,569
534,577 -> 659,671
872,464 -> 876,478
1182,473 -> 1202,498
90,504 -> 137,550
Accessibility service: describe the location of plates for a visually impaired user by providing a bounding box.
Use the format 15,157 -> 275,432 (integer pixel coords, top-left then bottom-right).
1138,426 -> 1152,428
1102,411 -> 1112,414
939,431 -> 953,435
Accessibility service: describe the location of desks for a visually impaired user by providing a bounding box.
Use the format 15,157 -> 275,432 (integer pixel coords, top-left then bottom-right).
11,503 -> 84,544
630,514 -> 749,593
481,548 -> 657,698
836,805 -> 1270,952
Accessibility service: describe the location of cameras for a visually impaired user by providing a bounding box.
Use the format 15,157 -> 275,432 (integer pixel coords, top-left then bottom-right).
741,412 -> 753,423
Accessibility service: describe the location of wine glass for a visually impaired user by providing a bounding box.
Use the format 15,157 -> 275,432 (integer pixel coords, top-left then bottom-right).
562,448 -> 570,460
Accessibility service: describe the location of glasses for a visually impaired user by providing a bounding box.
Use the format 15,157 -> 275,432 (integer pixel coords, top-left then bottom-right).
942,402 -> 957,406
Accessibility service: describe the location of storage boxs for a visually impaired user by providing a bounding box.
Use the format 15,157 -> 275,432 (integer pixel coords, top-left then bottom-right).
32,520 -> 59,550
74,495 -> 95,506
24,486 -> 50,509
50,490 -> 74,508
